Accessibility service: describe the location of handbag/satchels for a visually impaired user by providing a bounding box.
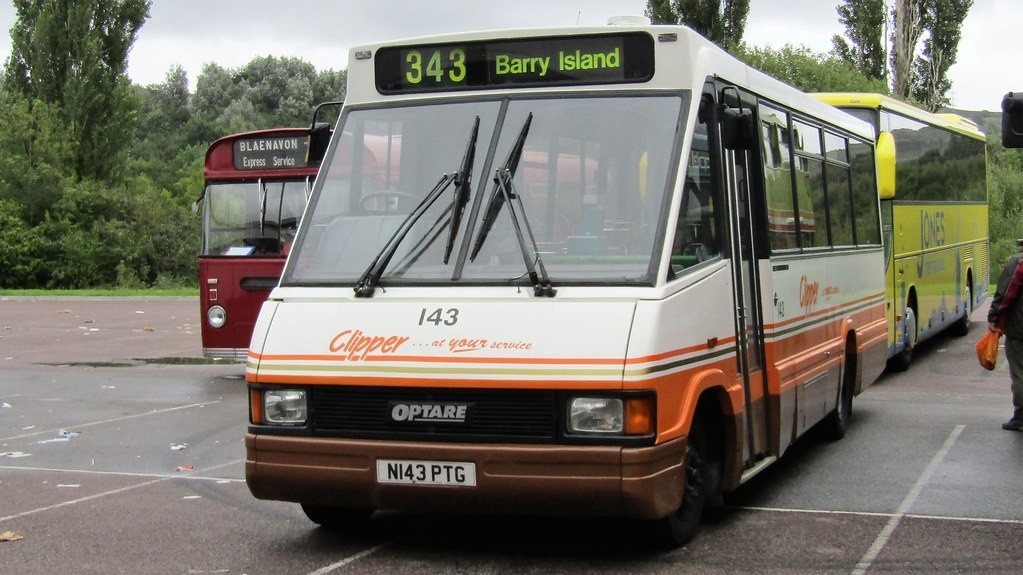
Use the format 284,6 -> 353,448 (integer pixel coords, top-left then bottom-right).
975,328 -> 1003,371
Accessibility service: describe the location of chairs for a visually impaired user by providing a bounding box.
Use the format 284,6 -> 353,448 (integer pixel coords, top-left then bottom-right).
308,215 -> 415,282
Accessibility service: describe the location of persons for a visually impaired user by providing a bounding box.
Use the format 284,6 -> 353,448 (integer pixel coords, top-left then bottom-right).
988,248 -> 1023,432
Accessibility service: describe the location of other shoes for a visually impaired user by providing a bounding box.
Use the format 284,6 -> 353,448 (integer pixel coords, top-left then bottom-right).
1002,409 -> 1023,430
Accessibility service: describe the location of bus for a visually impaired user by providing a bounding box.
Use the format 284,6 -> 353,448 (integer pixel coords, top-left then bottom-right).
245,22 -> 889,550
639,136 -> 816,254
192,126 -> 609,359
766,93 -> 990,375
